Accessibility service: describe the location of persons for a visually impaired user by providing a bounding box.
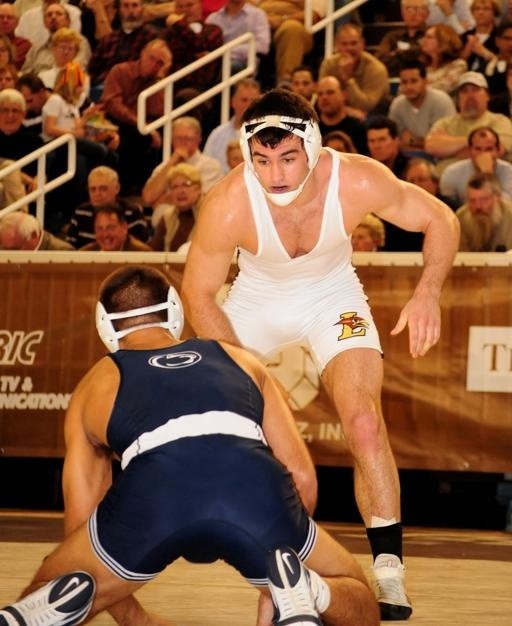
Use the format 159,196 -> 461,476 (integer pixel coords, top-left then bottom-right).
1,264 -> 380,625
178,89 -> 461,619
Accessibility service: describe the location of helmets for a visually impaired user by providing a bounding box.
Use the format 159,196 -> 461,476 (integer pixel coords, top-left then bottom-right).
454,72 -> 486,87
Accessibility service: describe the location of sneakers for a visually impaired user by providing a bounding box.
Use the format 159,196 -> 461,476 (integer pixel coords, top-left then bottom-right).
371,553 -> 413,618
1,571 -> 95,624
267,545 -> 322,626
